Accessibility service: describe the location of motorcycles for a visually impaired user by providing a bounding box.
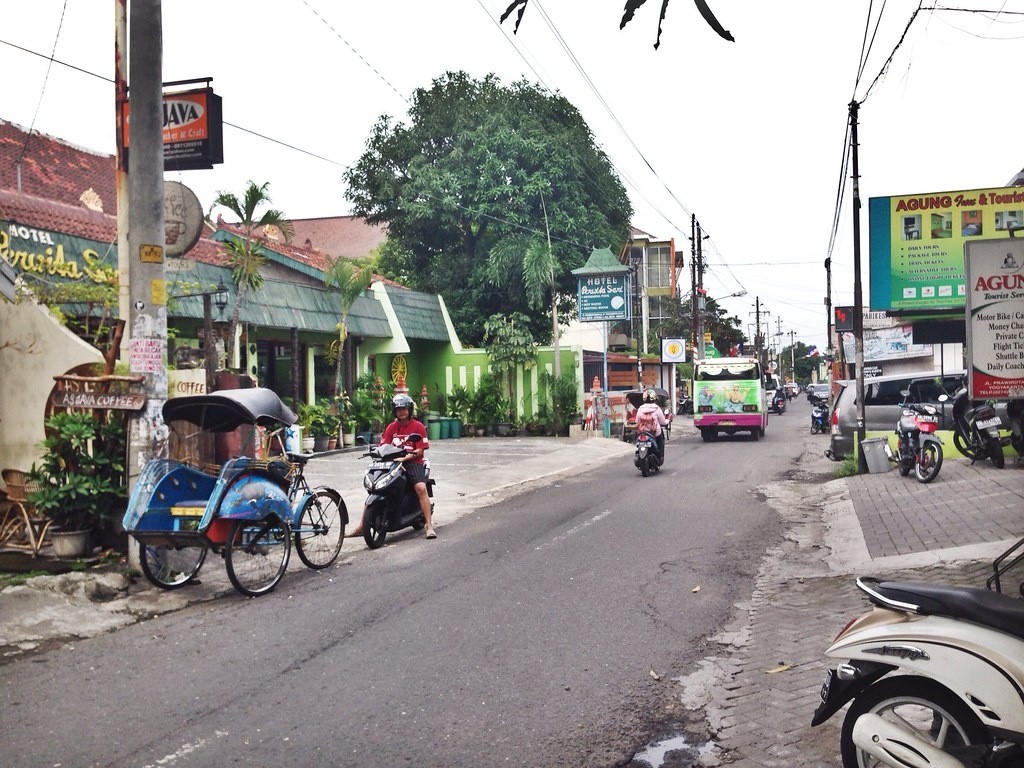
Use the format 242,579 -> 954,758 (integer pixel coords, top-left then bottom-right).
938,374 -> 1012,469
884,390 -> 948,483
810,398 -> 829,434
678,390 -> 694,415
774,395 -> 786,415
810,575 -> 1024,768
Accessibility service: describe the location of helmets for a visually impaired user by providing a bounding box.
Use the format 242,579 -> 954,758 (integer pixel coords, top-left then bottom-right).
642,389 -> 656,401
391,393 -> 414,421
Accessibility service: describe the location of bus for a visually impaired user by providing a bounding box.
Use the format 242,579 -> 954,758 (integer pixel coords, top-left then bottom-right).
693,357 -> 768,442
765,374 -> 786,409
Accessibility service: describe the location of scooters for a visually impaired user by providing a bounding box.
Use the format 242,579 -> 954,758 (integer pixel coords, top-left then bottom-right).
356,434 -> 436,549
634,409 -> 669,477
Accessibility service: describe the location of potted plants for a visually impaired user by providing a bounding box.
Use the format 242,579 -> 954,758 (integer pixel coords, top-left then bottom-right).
294,370 -> 584,454
22,411 -> 140,560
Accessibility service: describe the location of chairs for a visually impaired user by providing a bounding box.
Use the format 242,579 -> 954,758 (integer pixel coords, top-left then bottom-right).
0,468 -> 57,559
171,452 -> 297,531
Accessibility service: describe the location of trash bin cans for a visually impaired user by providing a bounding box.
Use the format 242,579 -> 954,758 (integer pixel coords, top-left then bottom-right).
860,434 -> 894,474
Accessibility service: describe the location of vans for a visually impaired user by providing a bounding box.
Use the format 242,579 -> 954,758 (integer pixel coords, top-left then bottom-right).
824,370 -> 1010,462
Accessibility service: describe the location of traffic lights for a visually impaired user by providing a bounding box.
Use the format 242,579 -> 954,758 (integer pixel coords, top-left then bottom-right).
834,306 -> 852,330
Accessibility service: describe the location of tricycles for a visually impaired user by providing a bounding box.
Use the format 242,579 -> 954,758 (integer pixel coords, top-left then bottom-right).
621,387 -> 671,442
122,388 -> 350,597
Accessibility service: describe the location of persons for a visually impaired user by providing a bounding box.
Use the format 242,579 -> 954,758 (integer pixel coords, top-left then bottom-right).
345,394 -> 437,539
772,385 -> 786,412
635,389 -> 673,461
787,387 -> 794,404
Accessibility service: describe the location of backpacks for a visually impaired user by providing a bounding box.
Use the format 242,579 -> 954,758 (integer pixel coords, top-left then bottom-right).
637,412 -> 657,436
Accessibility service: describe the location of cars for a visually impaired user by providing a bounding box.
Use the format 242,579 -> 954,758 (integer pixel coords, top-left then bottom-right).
784,382 -> 806,397
806,383 -> 830,406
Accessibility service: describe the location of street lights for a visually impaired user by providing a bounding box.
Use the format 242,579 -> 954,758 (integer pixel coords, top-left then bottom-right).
753,332 -> 784,362
698,291 -> 747,361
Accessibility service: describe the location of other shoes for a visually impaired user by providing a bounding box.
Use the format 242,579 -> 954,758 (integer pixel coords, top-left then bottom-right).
424,523 -> 437,538
344,529 -> 365,537
